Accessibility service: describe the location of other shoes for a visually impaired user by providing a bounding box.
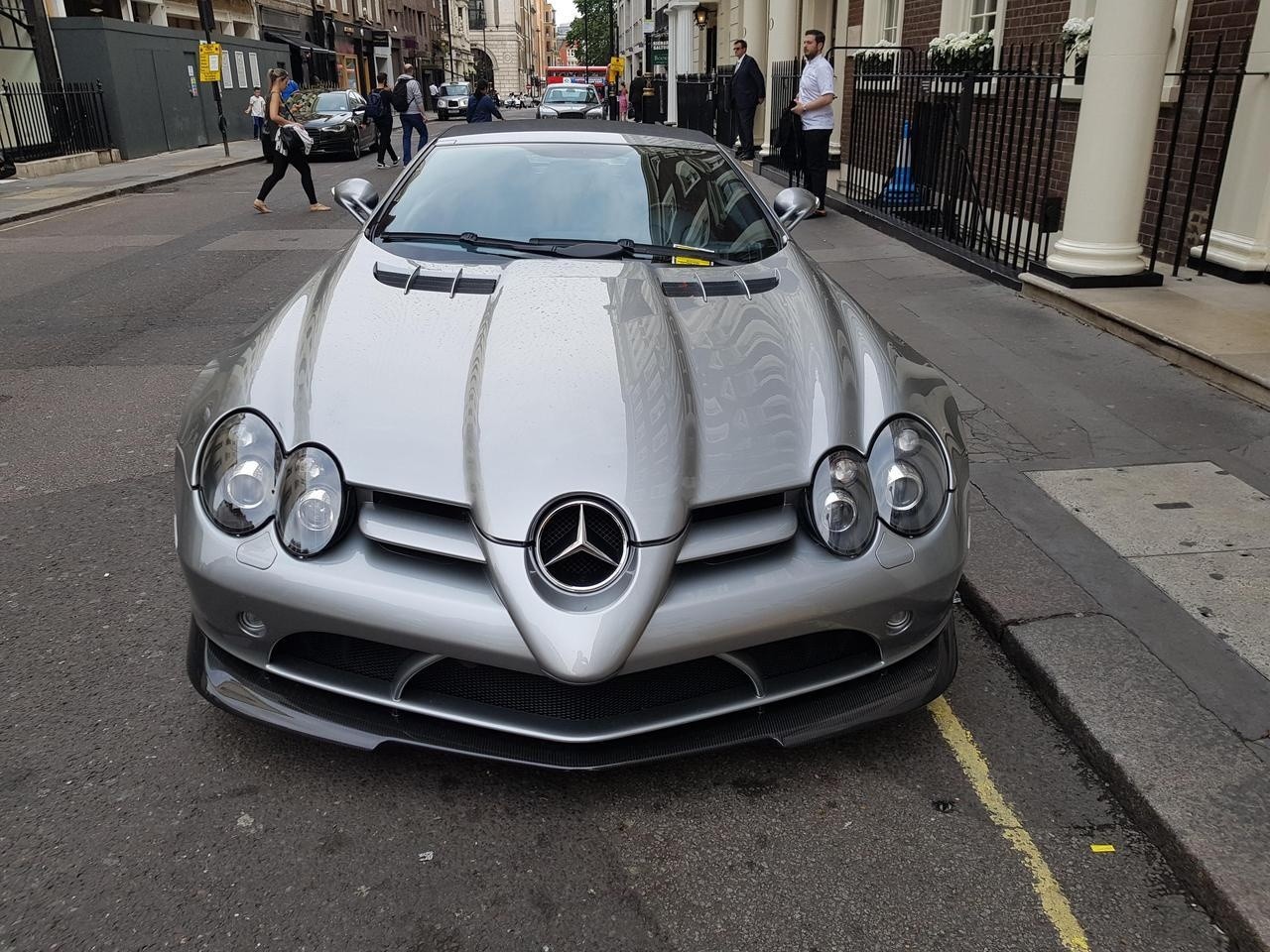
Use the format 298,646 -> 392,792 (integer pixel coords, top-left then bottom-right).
738,149 -> 755,160
809,210 -> 827,217
254,137 -> 257,141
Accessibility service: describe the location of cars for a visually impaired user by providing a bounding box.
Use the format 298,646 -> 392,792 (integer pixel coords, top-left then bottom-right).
536,83 -> 603,120
260,87 -> 379,162
505,94 -> 535,109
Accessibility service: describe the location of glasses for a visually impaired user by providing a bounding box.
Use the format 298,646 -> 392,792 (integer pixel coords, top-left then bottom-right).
733,47 -> 739,50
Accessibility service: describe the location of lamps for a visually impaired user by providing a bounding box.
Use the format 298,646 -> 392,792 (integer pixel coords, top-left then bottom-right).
692,3 -> 717,30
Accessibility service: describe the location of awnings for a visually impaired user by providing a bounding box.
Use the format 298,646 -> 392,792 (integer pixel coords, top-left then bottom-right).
264,32 -> 337,54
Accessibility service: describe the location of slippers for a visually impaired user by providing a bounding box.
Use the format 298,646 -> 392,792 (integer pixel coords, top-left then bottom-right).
310,204 -> 331,211
253,203 -> 273,213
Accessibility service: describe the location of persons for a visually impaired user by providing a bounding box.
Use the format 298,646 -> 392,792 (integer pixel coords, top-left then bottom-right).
620,69 -> 647,123
731,40 -> 766,161
394,63 -> 428,168
429,82 -> 439,114
466,79 -> 506,124
505,92 -> 528,110
252,67 -> 331,214
279,76 -> 299,104
361,72 -> 401,170
790,30 -> 834,220
245,87 -> 266,142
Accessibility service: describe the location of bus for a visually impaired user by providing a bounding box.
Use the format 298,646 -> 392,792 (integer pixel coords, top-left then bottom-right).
544,65 -> 608,100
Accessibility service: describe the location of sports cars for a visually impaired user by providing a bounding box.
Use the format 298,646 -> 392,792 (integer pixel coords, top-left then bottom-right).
168,112 -> 978,777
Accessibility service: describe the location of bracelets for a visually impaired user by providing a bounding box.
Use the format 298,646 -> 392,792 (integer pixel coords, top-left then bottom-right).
803,106 -> 806,113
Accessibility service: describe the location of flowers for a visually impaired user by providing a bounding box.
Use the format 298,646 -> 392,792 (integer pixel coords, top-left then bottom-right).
927,29 -> 995,65
853,39 -> 896,62
1059,16 -> 1095,62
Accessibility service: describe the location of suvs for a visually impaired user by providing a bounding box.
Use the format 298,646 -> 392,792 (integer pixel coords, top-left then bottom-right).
435,82 -> 473,120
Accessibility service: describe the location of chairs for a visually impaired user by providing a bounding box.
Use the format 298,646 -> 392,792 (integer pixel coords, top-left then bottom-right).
579,92 -> 592,99
553,90 -> 563,98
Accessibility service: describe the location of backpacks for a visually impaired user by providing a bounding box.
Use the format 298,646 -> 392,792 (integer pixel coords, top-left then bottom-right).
366,89 -> 389,120
390,78 -> 415,112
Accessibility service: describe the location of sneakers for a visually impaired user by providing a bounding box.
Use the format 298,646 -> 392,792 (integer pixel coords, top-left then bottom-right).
393,156 -> 401,166
378,163 -> 390,169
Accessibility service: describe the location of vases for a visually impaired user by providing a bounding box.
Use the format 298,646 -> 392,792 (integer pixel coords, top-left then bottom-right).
1074,57 -> 1088,85
935,56 -> 992,74
856,63 -> 893,75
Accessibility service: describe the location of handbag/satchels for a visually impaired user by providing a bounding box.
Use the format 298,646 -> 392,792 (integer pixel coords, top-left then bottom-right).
466,105 -> 476,123
272,127 -> 305,163
260,120 -> 270,142
